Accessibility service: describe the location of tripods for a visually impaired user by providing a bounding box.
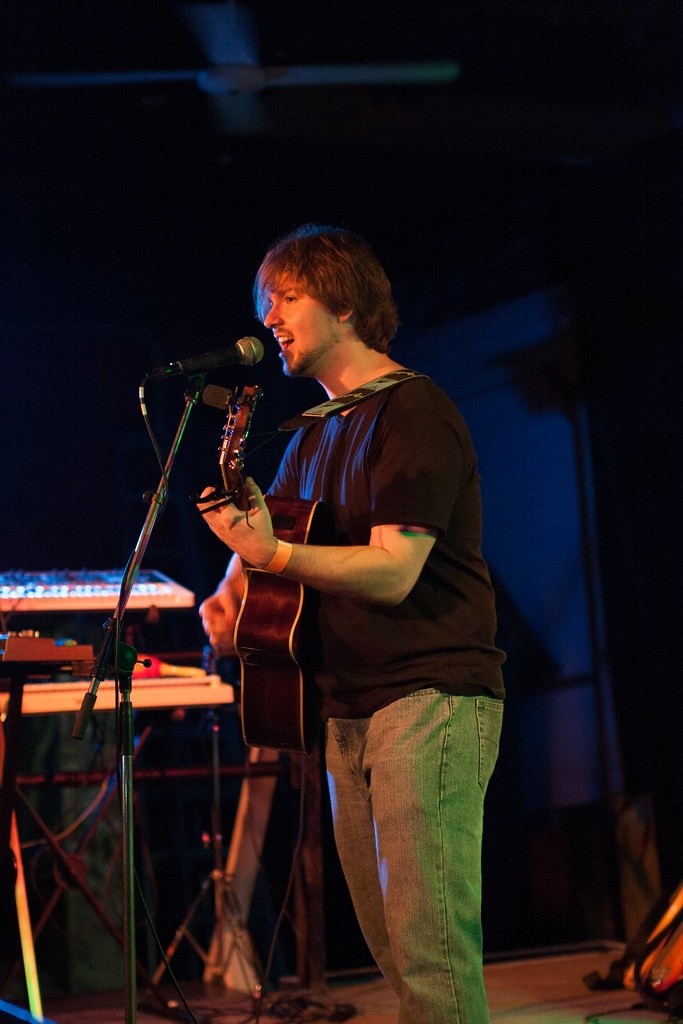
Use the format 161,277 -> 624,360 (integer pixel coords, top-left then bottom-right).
148,709 -> 279,1012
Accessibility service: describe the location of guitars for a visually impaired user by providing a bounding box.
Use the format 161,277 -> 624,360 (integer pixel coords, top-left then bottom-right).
622,879 -> 683,1006
217,385 -> 326,754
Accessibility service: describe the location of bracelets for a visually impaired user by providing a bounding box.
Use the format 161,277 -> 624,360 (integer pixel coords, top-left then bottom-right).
259,538 -> 293,574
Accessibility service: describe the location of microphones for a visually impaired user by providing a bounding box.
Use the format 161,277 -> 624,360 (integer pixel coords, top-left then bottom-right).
154,337 -> 264,376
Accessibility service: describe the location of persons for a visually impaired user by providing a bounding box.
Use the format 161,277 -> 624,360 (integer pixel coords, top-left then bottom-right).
197,226 -> 508,1017
